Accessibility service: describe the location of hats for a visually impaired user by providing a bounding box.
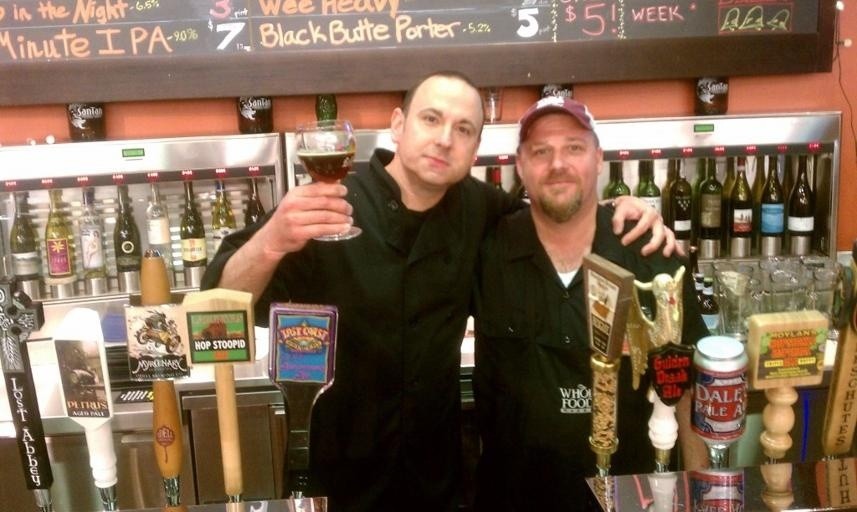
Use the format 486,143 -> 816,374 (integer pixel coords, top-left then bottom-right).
519,97 -> 601,147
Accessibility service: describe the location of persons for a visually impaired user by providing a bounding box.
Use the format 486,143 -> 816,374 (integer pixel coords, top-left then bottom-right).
199,69 -> 686,512
475,95 -> 711,512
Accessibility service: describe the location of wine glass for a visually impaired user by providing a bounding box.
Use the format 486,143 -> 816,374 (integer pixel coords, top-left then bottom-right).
294,118 -> 364,243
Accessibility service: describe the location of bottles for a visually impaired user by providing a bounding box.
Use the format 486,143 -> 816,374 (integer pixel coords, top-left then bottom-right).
78,187 -> 107,279
44,189 -> 75,278
759,155 -> 784,237
244,177 -> 267,228
632,160 -> 662,216
602,159 -> 630,200
145,182 -> 174,269
113,185 -> 142,270
485,166 -> 505,192
752,153 -> 766,207
9,188 -> 43,280
660,160 -> 676,224
631,158 -> 646,201
688,470 -> 745,512
693,273 -> 704,305
787,157 -> 815,236
781,153 -> 795,212
212,177 -> 238,255
687,247 -> 699,282
0,218 -> 8,285
689,336 -> 748,442
514,183 -> 532,205
697,277 -> 720,329
601,162 -> 616,200
723,156 -> 737,207
179,181 -> 208,267
727,156 -> 754,237
694,158 -> 707,203
667,155 -> 695,241
698,157 -> 723,239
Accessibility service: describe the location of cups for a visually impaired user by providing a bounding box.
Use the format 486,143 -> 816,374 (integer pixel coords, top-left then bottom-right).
712,251 -> 845,338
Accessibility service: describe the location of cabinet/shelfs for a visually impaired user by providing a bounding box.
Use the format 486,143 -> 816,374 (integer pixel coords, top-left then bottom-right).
2,108 -> 842,324
